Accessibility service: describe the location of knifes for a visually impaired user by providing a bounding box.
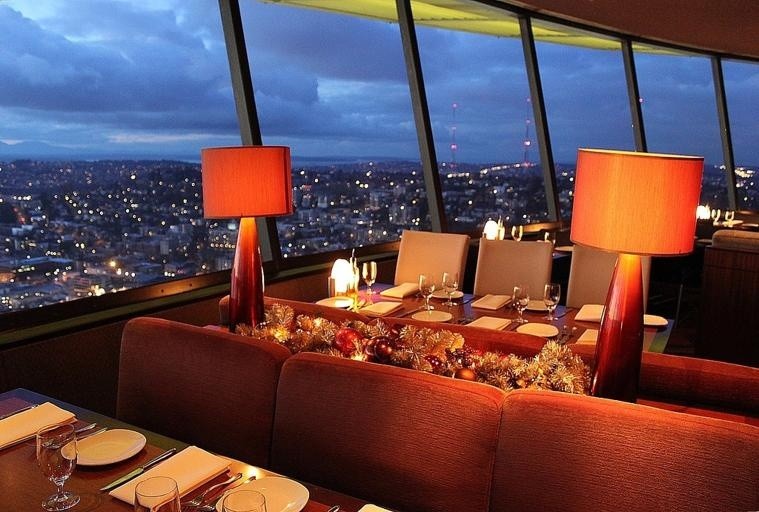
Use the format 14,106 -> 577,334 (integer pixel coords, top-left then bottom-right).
398,307 -> 418,318
459,296 -> 475,306
557,306 -> 574,318
557,324 -> 577,338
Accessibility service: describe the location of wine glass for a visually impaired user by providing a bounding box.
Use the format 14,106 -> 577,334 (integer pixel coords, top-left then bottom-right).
440,290 -> 457,306
34,425 -> 268,512
511,224 -> 556,245
418,289 -> 435,310
363,261 -> 561,323
712,209 -> 734,227
543,290 -> 561,323
511,289 -> 530,324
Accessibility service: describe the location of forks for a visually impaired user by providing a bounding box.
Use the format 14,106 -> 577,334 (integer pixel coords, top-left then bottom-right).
25,423 -> 107,450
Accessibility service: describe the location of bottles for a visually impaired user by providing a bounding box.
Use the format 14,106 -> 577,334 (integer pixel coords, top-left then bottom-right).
495,220 -> 505,241
347,257 -> 360,298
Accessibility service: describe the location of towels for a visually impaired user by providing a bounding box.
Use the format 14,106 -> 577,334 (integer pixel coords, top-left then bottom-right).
470,294 -> 512,310
575,328 -> 598,345
357,503 -> 391,511
109,446 -> 231,508
575,304 -> 604,322
380,282 -> 420,298
0,400 -> 78,449
359,301 -> 403,318
464,316 -> 511,330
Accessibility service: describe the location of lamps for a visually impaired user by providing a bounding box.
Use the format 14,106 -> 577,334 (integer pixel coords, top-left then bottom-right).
569,146 -> 703,405
200,146 -> 294,334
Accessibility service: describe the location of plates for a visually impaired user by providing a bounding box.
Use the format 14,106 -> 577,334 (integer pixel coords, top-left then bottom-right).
517,323 -> 559,338
641,312 -> 668,327
555,245 -> 572,252
414,309 -> 454,323
525,298 -> 552,311
318,296 -> 363,308
214,476 -> 309,512
733,219 -> 759,226
433,289 -> 464,298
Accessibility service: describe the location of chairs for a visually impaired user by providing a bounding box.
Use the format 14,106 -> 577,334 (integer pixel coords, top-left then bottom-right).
487,386 -> 759,512
474,238 -> 554,302
393,231 -> 471,291
266,351 -> 505,512
115,316 -> 290,469
566,243 -> 652,316
705,229 -> 759,307
512,220 -> 564,233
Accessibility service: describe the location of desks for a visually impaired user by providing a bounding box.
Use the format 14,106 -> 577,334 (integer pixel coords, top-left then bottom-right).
0,387 -> 396,512
312,282 -> 673,354
